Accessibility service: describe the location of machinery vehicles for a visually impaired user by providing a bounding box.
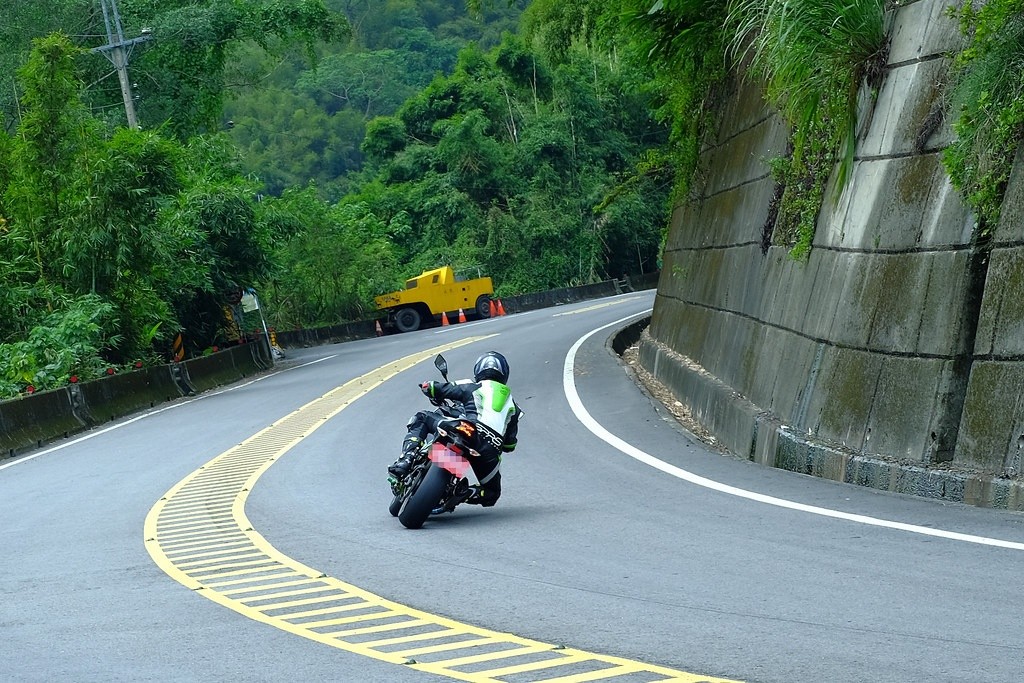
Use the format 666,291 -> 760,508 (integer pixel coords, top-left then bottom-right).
373,265 -> 496,333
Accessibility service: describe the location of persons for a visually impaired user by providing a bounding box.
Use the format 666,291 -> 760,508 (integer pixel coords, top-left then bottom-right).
388,351 -> 521,507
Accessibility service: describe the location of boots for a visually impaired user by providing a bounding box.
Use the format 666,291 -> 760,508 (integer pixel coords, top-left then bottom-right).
387,433 -> 424,480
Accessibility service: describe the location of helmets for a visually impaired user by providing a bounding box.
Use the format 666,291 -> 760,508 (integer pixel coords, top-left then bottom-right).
473,350 -> 509,378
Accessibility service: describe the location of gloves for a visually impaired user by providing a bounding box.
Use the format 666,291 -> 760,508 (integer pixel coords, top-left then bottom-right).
422,380 -> 430,392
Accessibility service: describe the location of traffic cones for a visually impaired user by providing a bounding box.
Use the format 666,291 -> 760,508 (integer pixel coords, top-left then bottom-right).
490,300 -> 498,318
458,307 -> 467,323
495,299 -> 507,316
375,320 -> 383,338
442,311 -> 450,326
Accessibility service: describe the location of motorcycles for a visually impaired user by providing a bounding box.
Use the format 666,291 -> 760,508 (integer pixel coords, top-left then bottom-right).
387,353 -> 525,530
